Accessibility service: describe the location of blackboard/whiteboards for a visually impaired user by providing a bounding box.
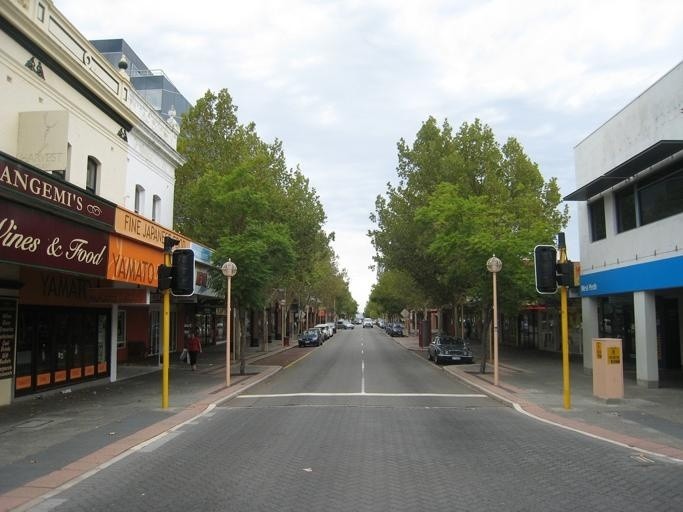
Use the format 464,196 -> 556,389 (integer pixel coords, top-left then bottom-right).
0,295 -> 19,380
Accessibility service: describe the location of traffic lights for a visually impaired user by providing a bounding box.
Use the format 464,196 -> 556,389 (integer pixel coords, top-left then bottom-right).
534,244 -> 558,294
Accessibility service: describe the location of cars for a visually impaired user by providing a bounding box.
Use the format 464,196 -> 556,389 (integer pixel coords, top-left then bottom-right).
338,320 -> 354,329
363,318 -> 373,328
427,336 -> 472,364
298,323 -> 336,348
373,319 -> 405,337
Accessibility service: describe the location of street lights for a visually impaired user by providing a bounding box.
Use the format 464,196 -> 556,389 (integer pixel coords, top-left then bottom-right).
486,254 -> 503,386
221,257 -> 239,388
281,299 -> 286,344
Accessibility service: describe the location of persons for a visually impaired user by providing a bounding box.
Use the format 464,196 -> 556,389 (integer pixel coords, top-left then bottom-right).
462,318 -> 470,339
184,332 -> 202,372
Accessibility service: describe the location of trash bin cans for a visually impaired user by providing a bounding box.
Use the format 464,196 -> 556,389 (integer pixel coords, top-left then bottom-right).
284,336 -> 289,347
268,336 -> 272,343
